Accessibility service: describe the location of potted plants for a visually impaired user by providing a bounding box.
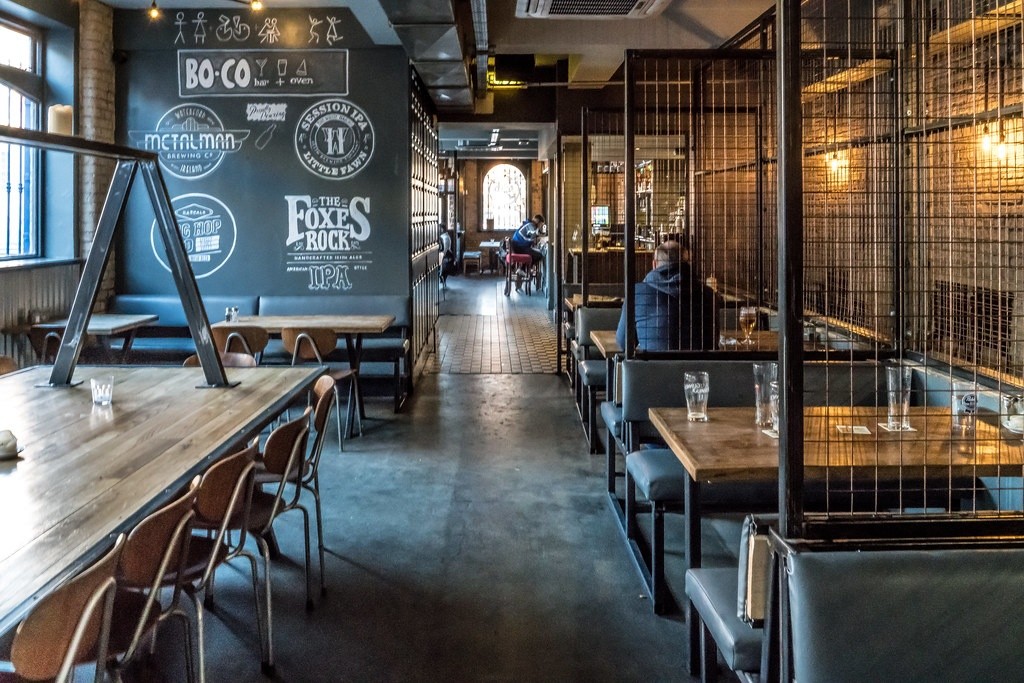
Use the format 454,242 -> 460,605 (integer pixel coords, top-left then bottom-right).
47,104 -> 73,135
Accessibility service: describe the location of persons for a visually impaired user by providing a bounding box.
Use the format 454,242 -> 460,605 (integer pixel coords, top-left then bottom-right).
616,241 -> 720,350
511,215 -> 544,277
439,223 -> 451,264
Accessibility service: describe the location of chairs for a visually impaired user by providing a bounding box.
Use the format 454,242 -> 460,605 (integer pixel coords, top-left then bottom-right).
504,237 -> 532,297
0,326 -> 364,683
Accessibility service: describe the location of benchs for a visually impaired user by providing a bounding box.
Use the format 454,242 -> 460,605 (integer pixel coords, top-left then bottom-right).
464,251 -> 483,277
88,294 -> 259,355
563,284 -> 1023,683
255,294 -> 412,414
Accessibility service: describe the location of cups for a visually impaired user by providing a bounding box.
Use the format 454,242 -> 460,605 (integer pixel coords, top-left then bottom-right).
770,381 -> 779,433
1000,395 -> 1023,435
950,381 -> 979,431
752,362 -> 778,427
886,367 -> 912,430
491,239 -> 494,244
90,375 -> 114,405
683,371 -> 709,422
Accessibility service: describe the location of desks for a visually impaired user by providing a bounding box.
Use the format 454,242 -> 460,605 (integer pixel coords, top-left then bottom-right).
565,294 -> 752,373
590,330 -> 873,403
479,241 -> 501,275
650,407 -> 1023,676
209,316 -> 396,440
0,366 -> 330,652
1,314 -> 159,367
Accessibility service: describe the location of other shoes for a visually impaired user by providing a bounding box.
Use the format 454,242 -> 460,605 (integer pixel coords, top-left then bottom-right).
516,268 -> 530,278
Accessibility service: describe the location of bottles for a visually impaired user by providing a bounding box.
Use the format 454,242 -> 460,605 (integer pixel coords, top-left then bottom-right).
231,307 -> 239,323
225,306 -> 231,323
706,275 -> 716,291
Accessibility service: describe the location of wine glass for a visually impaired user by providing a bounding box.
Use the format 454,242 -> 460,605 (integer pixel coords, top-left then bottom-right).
739,307 -> 757,345
